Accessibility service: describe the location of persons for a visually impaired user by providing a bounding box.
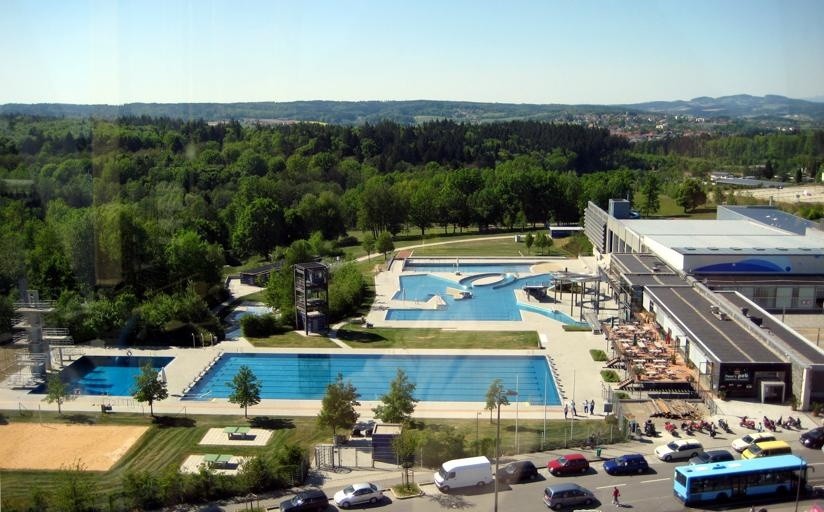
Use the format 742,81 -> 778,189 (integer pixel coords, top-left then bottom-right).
570,400 -> 578,416
709,422 -> 716,438
588,400 -> 595,415
611,487 -> 621,507
757,422 -> 762,432
564,404 -> 569,418
582,400 -> 590,414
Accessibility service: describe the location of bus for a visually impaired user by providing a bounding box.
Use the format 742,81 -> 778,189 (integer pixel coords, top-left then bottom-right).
672,451 -> 816,508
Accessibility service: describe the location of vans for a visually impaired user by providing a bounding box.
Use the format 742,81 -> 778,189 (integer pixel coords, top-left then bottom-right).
542,482 -> 599,510
432,455 -> 492,494
739,439 -> 793,461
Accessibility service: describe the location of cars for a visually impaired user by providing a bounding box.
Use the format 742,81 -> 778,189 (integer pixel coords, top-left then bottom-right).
334,481 -> 384,509
799,425 -> 824,450
547,453 -> 590,477
603,453 -> 649,477
730,431 -> 776,452
687,449 -> 735,465
498,461 -> 540,484
280,488 -> 330,511
654,438 -> 704,462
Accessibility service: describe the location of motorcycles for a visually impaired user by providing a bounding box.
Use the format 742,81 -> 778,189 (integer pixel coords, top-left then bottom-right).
626,416 -> 802,437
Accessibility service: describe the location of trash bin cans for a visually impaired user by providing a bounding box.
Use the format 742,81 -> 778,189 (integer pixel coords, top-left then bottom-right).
597,450 -> 601,456
